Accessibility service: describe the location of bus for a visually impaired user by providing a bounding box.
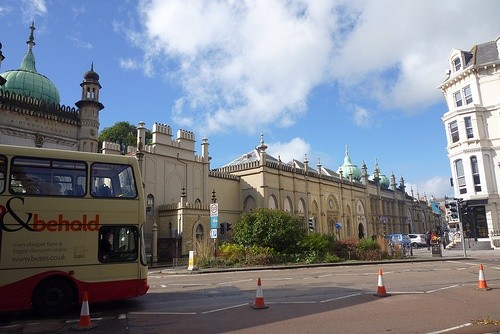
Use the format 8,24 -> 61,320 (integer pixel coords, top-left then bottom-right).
0,144 -> 150,320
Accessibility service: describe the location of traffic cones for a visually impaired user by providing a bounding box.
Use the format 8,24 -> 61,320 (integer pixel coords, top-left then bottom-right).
70,290 -> 98,331
475,264 -> 492,291
373,268 -> 392,297
250,276 -> 270,310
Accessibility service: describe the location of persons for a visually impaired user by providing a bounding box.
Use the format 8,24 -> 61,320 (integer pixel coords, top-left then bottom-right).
441,231 -> 447,249
425,231 -> 431,251
98,230 -> 122,257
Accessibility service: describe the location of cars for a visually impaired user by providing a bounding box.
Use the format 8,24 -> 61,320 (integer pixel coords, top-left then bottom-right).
385,233 -> 442,249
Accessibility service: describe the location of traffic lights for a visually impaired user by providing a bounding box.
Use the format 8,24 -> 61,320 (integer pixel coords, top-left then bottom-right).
308,217 -> 315,232
459,201 -> 470,221
449,200 -> 460,223
